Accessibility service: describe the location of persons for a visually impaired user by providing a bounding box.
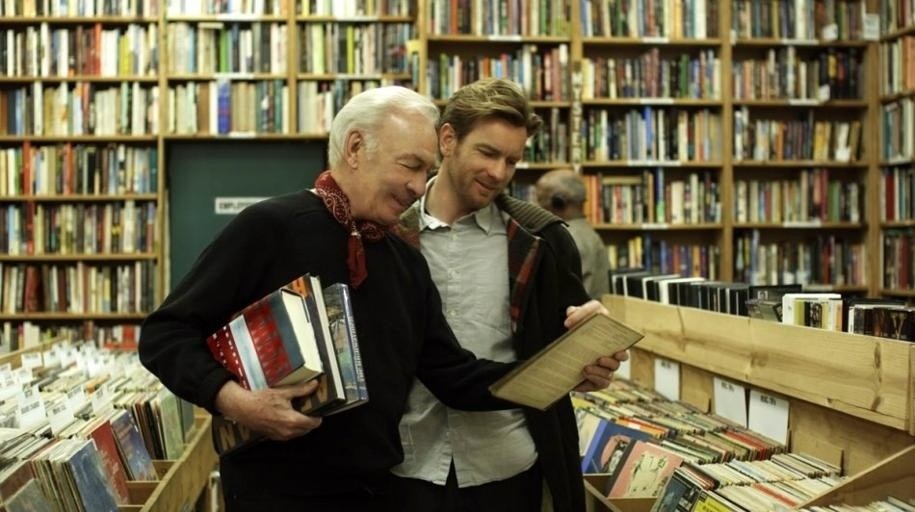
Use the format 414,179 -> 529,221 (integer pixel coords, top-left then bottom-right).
137,84 -> 631,512
387,77 -> 612,511
535,166 -> 614,306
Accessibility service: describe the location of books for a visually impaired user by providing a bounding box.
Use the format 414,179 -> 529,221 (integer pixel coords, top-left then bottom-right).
579,0 -> 720,162
585,165 -> 720,276
0,80 -> 159,196
165,0 -> 418,137
608,267 -> 914,344
733,105 -> 866,286
428,1 -> 573,208
0,339 -> 195,512
880,99 -> 914,292
488,310 -> 644,412
2,202 -> 160,352
1,1 -> 157,76
729,1 -> 914,99
569,375 -> 915,511
203,273 -> 369,462
